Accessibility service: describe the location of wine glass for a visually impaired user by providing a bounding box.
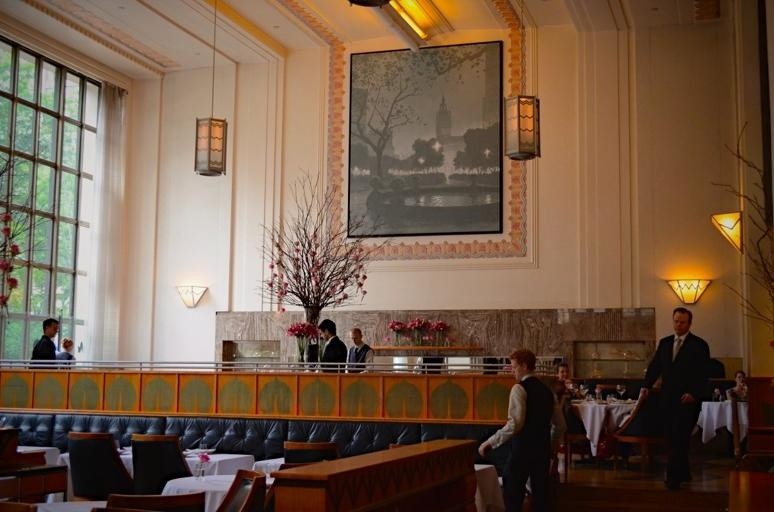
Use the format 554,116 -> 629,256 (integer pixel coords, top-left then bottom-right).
617,385 -> 626,404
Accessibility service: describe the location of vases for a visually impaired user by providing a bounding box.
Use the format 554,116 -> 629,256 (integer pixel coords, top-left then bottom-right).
294,337 -> 311,364
393,332 -> 446,346
304,304 -> 321,344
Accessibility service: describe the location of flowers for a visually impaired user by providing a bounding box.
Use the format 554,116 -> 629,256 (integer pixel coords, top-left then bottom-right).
388,318 -> 449,332
286,321 -> 319,361
1,153 -> 46,311
257,169 -> 370,321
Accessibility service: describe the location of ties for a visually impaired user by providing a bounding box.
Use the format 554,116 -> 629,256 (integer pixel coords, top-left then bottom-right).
673,338 -> 681,360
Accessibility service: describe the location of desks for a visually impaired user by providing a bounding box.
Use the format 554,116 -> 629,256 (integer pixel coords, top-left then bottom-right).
474,463 -> 506,512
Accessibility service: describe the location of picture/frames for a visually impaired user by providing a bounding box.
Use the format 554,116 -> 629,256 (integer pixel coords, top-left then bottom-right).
349,40 -> 504,238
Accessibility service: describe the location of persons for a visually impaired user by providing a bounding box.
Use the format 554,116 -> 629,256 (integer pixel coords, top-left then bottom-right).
551,364 -> 586,434
639,307 -> 710,491
345,327 -> 373,373
478,350 -> 566,512
413,357 -> 446,374
55,337 -> 76,369
29,319 -> 59,369
317,319 -> 347,373
726,371 -> 748,401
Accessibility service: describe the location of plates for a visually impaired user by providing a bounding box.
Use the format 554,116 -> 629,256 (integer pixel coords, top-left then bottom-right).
572,400 -> 584,402
192,448 -> 216,453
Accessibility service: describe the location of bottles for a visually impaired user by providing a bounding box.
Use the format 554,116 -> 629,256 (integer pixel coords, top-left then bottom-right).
535,360 -> 558,377
595,384 -> 603,403
579,385 -> 584,399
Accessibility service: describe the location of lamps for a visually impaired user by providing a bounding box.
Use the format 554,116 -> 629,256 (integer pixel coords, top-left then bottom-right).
193,0 -> 229,177
663,275 -> 713,304
503,0 -> 541,161
177,285 -> 207,308
710,211 -> 743,254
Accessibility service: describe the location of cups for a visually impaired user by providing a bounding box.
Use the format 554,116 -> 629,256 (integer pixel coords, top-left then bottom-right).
607,394 -> 614,404
586,394 -> 592,401
200,444 -> 207,457
